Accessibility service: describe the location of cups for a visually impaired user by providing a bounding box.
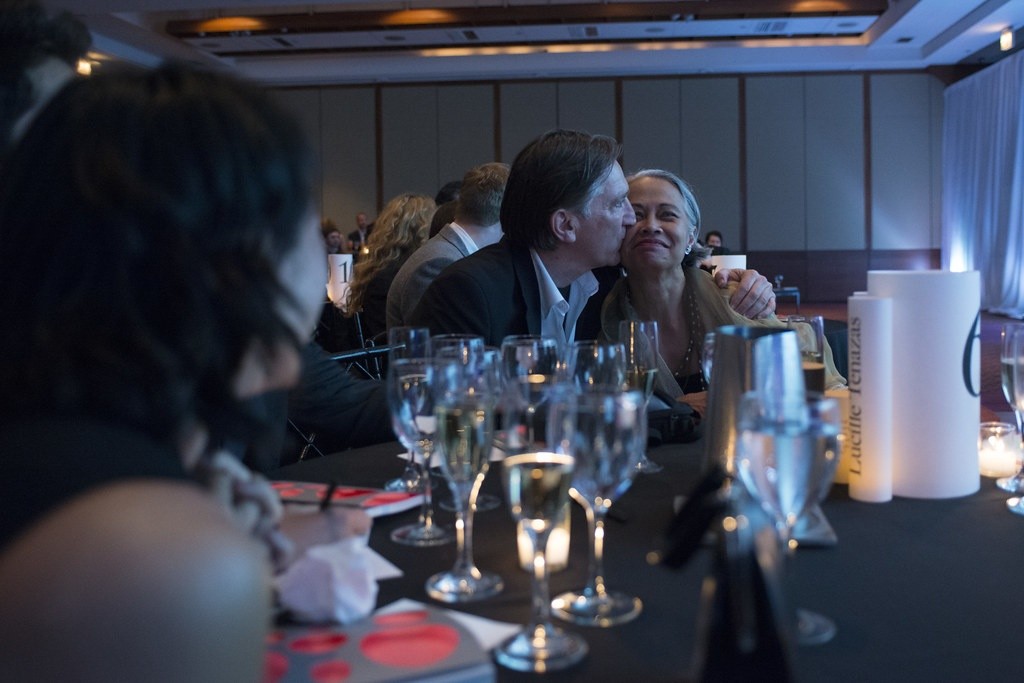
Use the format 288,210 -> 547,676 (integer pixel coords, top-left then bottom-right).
787,315 -> 826,398
980,422 -> 1017,477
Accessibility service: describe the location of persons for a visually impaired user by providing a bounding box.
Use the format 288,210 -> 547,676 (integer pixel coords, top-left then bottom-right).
0,53 -> 775,682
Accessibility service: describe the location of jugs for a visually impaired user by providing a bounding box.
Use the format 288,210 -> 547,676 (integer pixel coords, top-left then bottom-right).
703,325 -> 812,530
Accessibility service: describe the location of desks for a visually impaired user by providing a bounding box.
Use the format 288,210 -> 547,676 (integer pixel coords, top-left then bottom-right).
766,273 -> 801,313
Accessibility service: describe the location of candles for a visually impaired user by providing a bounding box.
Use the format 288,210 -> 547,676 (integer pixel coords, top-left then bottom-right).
979,436 -> 1022,478
518,528 -> 568,571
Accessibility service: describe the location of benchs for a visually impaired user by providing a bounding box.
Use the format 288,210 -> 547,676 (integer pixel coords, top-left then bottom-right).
746,247 -> 941,324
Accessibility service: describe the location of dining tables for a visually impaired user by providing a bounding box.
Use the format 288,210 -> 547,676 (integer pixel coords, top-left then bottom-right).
259,408 -> 1024,683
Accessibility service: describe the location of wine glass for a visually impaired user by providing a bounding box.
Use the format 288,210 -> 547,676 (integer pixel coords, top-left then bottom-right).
387,319 -> 657,666
733,389 -> 836,643
995,323 -> 1024,514
774,275 -> 783,288
